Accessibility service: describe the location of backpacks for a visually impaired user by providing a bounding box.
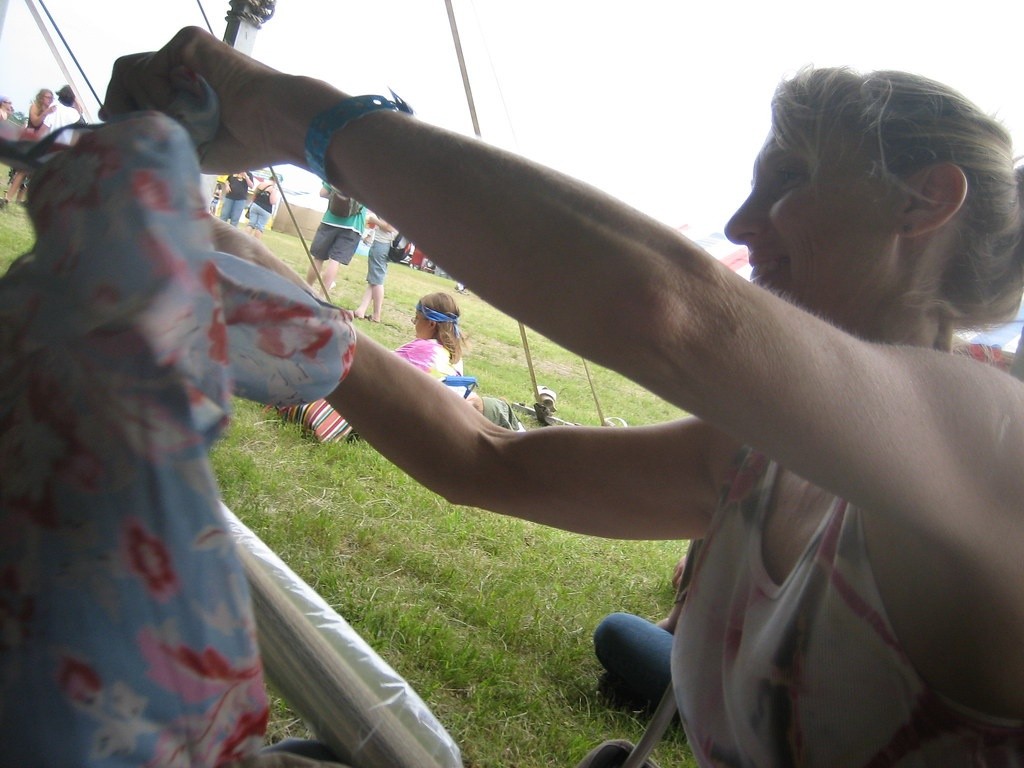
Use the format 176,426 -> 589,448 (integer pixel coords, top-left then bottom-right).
328,190 -> 363,218
388,233 -> 411,263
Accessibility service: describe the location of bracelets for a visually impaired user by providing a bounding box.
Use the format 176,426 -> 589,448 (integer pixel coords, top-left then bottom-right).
304,87 -> 415,185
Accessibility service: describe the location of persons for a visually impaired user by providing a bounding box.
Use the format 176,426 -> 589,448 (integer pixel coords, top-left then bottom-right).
99,26 -> 1024,768
306,180 -> 398,323
6,86 -> 81,203
0,95 -> 13,122
245,176 -> 279,239
362,214 -> 467,290
210,184 -> 222,215
593,539 -> 704,703
390,291 -> 464,382
219,171 -> 254,226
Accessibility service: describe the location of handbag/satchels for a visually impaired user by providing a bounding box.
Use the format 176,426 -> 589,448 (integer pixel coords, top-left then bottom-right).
245,208 -> 250,219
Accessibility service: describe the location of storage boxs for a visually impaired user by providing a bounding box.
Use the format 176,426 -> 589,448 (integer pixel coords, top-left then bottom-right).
272,200 -> 324,240
